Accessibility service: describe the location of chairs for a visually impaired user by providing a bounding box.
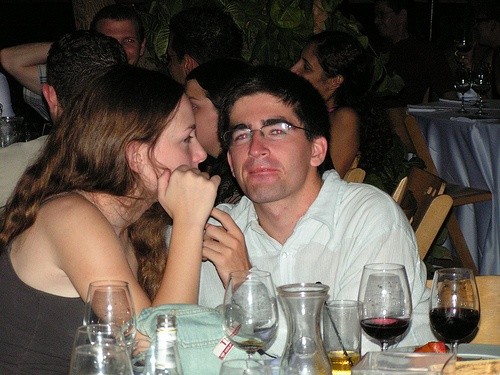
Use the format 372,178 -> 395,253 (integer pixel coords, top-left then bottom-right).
390,165 -> 454,259
389,106 -> 493,277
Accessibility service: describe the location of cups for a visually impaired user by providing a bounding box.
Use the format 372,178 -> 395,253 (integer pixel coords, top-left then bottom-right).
321,299 -> 364,374
0,116 -> 26,147
220,359 -> 273,375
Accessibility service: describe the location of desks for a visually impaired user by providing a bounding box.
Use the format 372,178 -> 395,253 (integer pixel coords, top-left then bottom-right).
403,91 -> 500,276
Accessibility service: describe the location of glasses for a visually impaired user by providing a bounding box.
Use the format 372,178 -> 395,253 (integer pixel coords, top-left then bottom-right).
220,121 -> 318,144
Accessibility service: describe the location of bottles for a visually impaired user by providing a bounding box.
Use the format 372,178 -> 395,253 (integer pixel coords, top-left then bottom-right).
144,315 -> 184,375
272,282 -> 334,375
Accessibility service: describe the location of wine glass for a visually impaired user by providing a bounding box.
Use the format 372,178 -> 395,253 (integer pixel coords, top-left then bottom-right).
357,261 -> 412,351
222,271 -> 279,360
453,65 -> 472,111
471,65 -> 491,115
429,268 -> 480,361
68,280 -> 136,375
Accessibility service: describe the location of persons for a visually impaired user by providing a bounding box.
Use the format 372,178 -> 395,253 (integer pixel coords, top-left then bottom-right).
374,0 -> 500,105
186,57 -> 243,206
168,5 -> 243,86
0,73 -> 15,116
198,68 -> 439,356
0,30 -> 130,208
0,3 -> 146,121
0,64 -> 221,375
290,29 -> 405,194
